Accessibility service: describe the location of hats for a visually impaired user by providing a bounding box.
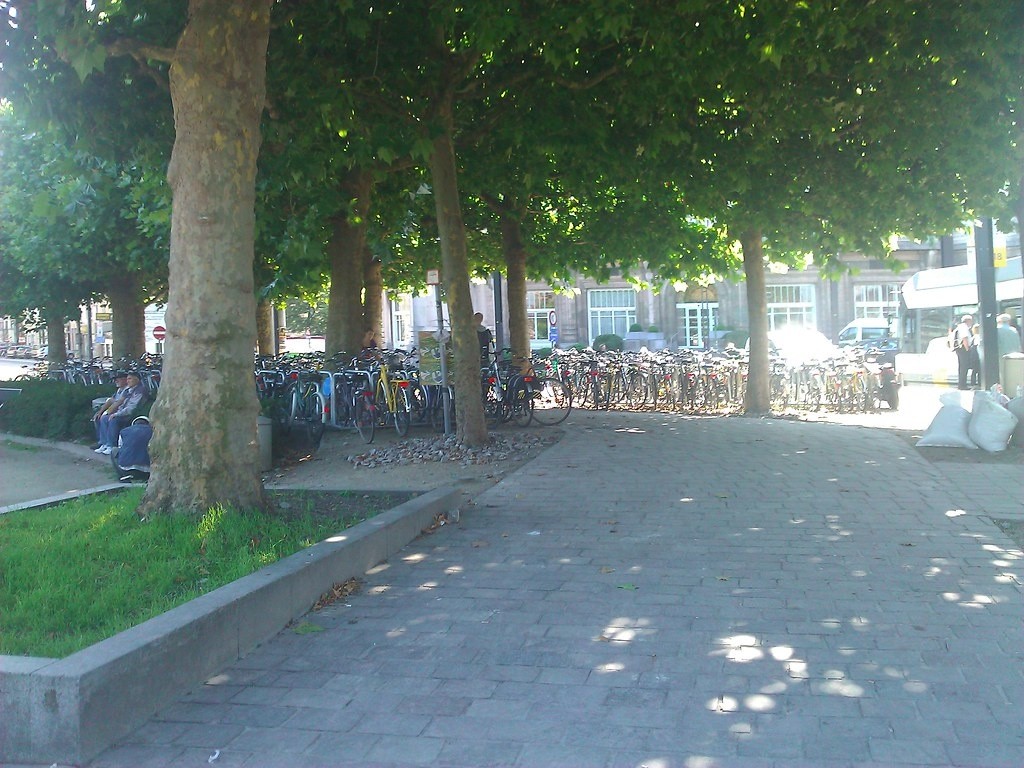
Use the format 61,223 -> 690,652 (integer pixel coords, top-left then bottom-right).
131,416 -> 149,426
113,371 -> 128,379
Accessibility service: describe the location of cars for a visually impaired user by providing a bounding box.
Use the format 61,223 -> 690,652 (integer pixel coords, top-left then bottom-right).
36,345 -> 75,361
16,347 -> 32,359
0,344 -> 8,358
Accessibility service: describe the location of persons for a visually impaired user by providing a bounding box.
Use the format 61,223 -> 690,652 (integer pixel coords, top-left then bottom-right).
997,314 -> 1021,385
369,328 -> 376,347
474,313 -> 492,372
91,372 -> 143,455
951,312 -> 981,390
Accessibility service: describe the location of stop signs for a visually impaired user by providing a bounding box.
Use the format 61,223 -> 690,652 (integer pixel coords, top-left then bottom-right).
152,325 -> 166,341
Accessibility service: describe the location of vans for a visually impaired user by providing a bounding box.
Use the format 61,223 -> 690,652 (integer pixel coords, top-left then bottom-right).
31,344 -> 45,360
836,315 -> 899,348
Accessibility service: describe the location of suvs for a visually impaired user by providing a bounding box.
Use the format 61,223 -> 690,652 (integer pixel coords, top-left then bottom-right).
6,346 -> 21,360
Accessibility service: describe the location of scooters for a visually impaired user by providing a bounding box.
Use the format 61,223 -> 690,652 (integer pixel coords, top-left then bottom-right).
875,345 -> 904,410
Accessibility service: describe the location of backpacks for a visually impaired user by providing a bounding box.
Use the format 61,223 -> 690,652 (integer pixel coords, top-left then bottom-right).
948,329 -> 963,351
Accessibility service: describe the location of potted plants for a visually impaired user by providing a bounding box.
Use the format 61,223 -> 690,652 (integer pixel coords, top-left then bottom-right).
645,325 -> 665,339
626,323 -> 645,340
709,325 -> 733,338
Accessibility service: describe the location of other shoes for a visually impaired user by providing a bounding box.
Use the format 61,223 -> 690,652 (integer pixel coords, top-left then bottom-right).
91,443 -> 102,449
960,386 -> 972,390
121,478 -> 132,483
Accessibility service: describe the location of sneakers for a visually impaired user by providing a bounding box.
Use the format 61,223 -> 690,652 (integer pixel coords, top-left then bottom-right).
95,445 -> 106,454
103,447 -> 112,454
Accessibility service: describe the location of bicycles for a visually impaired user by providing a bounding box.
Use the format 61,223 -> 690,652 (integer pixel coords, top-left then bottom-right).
13,341 -> 880,445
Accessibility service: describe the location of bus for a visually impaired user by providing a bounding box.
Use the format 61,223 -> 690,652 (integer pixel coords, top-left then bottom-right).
892,256 -> 1024,386
